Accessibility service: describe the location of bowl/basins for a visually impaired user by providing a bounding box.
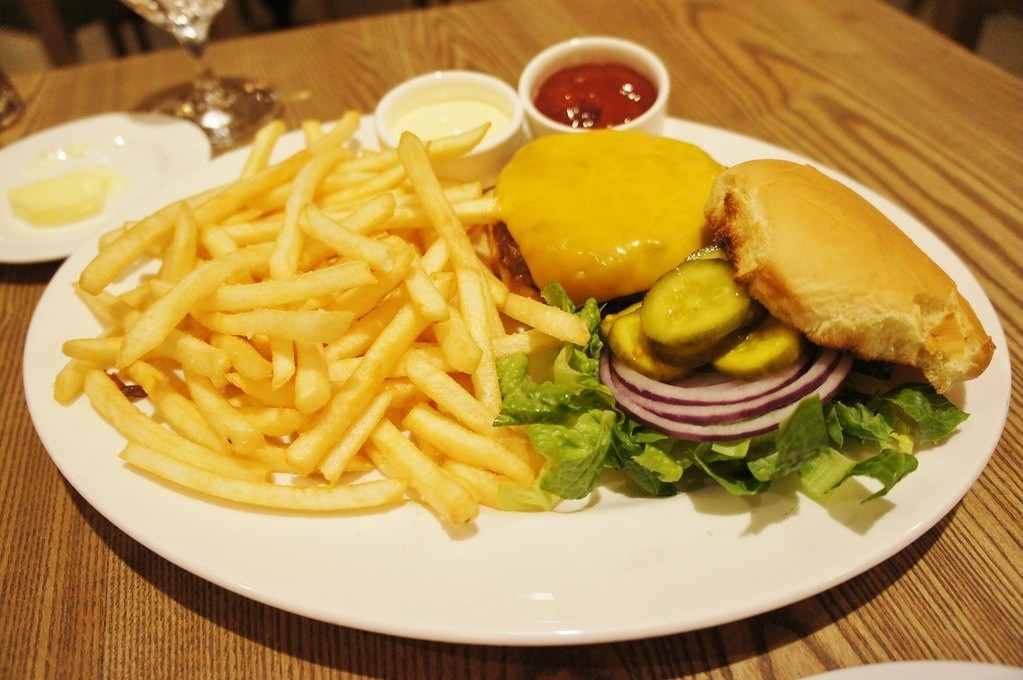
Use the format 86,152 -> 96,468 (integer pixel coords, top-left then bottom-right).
518,37 -> 671,139
375,71 -> 526,190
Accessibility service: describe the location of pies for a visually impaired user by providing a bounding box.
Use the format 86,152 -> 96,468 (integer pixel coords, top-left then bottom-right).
705,159 -> 990,383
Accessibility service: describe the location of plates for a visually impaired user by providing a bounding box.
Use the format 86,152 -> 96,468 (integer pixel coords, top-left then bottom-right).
23,115 -> 1014,647
0,113 -> 212,265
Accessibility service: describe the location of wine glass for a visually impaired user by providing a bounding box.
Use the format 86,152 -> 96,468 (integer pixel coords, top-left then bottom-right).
118,0 -> 280,148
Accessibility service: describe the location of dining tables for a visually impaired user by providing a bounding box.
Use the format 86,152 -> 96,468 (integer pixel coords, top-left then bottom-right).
0,0 -> 1022,680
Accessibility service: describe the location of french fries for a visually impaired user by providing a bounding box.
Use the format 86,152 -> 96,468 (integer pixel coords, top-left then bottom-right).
54,112 -> 591,528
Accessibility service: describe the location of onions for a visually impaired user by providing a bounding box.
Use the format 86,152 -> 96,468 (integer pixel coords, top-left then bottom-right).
597,343 -> 854,440
